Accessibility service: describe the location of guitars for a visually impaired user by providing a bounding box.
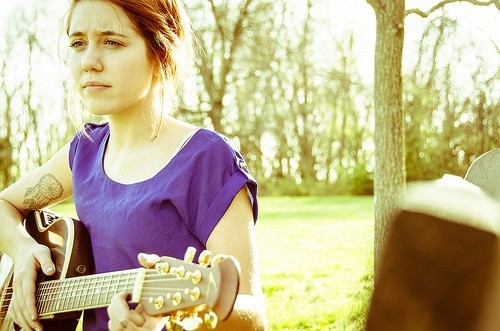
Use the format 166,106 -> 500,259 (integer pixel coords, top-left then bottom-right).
0,208 -> 241,330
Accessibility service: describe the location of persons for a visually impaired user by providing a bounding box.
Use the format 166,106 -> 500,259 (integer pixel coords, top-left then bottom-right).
0,0 -> 269,331
